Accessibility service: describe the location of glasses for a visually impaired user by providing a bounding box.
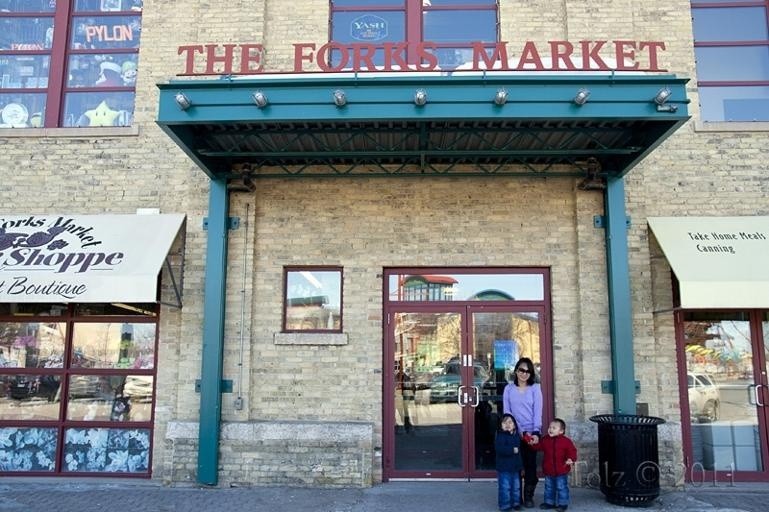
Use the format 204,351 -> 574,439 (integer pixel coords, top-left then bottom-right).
519,368 -> 530,373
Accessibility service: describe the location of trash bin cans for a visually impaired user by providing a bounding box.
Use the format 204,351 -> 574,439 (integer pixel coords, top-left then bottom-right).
589,414 -> 665,507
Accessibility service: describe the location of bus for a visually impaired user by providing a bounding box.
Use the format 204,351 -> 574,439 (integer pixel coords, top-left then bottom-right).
285,294 -> 330,330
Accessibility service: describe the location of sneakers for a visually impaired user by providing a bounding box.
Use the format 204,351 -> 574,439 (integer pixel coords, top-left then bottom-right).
503,496 -> 568,512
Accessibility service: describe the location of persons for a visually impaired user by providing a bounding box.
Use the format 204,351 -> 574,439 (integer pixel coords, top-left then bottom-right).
494,413 -> 531,512
503,358 -> 543,508
522,418 -> 577,512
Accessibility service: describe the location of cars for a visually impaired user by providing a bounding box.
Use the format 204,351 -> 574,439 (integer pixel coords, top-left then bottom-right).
6,363 -> 154,403
394,356 -> 490,404
688,372 -> 720,424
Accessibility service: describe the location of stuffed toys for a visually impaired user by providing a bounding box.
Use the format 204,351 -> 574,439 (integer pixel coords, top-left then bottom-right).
68,54 -> 136,126
